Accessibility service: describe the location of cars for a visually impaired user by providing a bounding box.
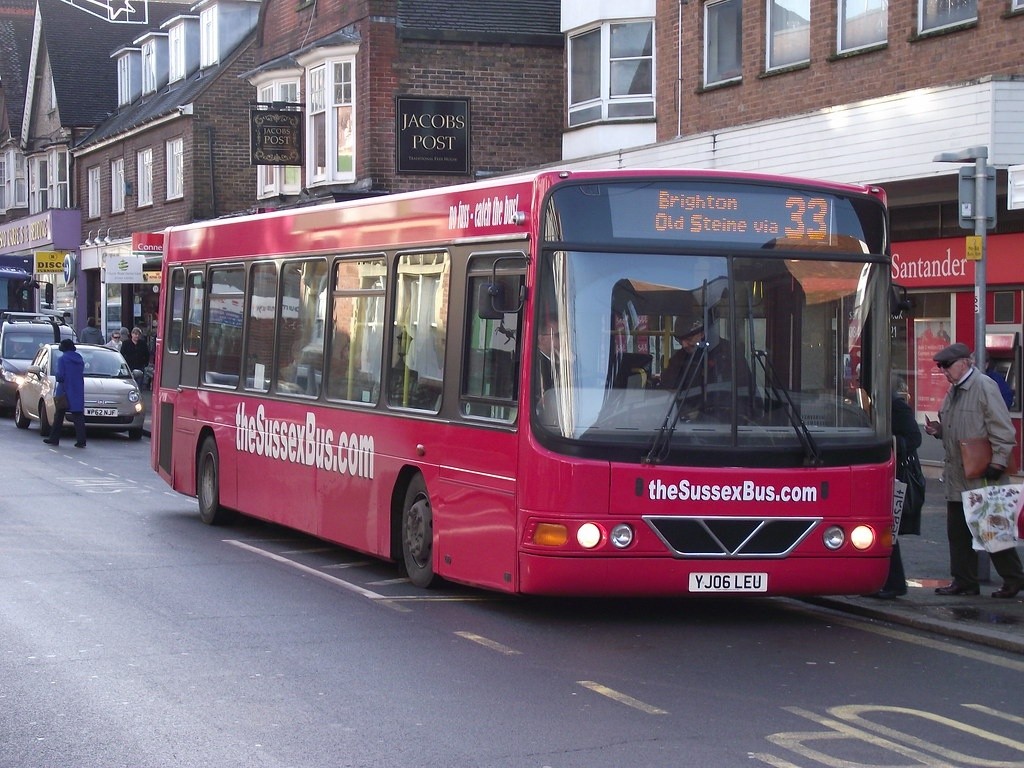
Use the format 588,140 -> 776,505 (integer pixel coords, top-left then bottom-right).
13,342 -> 146,440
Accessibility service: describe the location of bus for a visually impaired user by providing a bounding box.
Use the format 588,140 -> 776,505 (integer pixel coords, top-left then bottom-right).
146,168 -> 913,610
0,253 -> 54,335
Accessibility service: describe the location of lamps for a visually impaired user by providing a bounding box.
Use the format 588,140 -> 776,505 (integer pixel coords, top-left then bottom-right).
104,227 -> 112,243
85,230 -> 93,247
94,228 -> 102,245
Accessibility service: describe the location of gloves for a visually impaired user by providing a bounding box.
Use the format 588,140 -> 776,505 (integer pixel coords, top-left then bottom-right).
985,467 -> 1002,480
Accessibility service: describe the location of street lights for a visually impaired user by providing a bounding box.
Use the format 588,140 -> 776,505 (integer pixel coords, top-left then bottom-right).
932,145 -> 990,377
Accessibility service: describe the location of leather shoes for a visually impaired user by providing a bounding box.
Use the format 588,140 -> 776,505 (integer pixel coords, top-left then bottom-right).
935,580 -> 981,595
992,583 -> 1024,598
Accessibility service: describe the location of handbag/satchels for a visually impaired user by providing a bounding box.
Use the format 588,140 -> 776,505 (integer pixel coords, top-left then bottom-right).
961,476 -> 1024,553
899,438 -> 926,535
53,393 -> 70,411
958,437 -> 1018,481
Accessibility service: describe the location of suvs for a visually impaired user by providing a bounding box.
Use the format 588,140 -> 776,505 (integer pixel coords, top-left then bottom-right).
0,310 -> 79,415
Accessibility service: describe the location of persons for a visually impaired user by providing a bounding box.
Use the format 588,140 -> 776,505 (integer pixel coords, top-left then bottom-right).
922,343 -> 1024,598
536,316 -> 561,407
652,314 -> 763,425
80,316 -> 104,345
863,368 -> 922,600
924,321 -> 950,341
107,326 -> 150,392
43,339 -> 87,447
63,312 -> 74,328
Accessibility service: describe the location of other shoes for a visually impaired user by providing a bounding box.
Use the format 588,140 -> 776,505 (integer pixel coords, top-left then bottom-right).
74,443 -> 86,448
878,590 -> 907,599
43,439 -> 59,445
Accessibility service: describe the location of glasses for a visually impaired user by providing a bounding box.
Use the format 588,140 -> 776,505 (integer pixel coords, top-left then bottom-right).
675,337 -> 692,343
542,332 -> 559,338
112,336 -> 120,338
936,360 -> 958,368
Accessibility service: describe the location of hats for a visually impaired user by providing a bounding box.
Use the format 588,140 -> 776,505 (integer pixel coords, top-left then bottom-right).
675,315 -> 713,338
933,343 -> 970,361
58,339 -> 76,351
